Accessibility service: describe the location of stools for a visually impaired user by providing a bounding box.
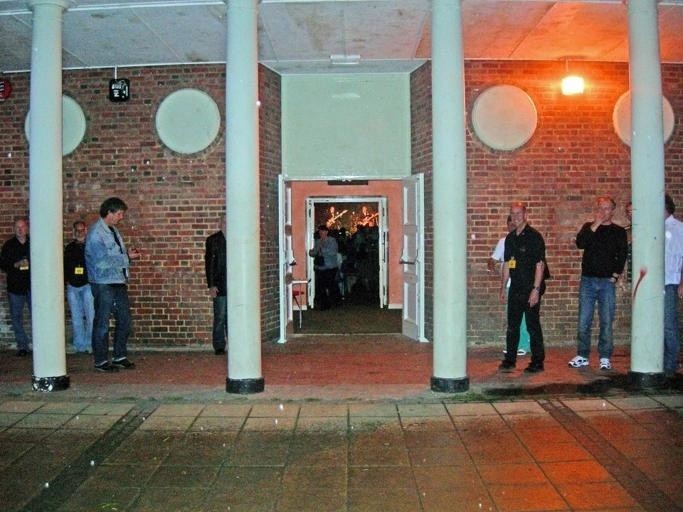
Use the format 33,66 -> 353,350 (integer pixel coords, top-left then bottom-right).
293,291 -> 304,309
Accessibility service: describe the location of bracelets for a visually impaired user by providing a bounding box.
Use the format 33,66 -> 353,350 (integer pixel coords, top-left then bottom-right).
612,276 -> 618,282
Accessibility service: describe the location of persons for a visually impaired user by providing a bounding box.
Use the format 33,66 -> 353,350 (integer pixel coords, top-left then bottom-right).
500,201 -> 550,376
120,81 -> 126,97
308,205 -> 378,310
665,194 -> 683,379
84,196 -> 142,372
488,216 -> 532,357
205,213 -> 227,355
623,202 -> 632,291
0,219 -> 31,356
64,221 -> 96,355
568,196 -> 627,370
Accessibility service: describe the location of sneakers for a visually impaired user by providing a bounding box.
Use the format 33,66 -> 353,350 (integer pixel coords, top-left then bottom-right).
600,358 -> 610,369
215,350 -> 225,355
18,350 -> 26,356
665,368 -> 673,378
95,362 -> 119,373
569,355 -> 589,367
499,360 -> 514,369
113,359 -> 135,370
524,363 -> 544,373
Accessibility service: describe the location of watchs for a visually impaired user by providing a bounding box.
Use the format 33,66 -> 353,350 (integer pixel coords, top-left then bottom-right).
533,286 -> 540,291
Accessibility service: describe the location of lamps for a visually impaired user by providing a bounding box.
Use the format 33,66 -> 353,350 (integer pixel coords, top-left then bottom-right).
562,75 -> 585,95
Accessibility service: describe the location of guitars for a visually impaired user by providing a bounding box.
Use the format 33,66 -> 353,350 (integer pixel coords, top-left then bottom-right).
327,210 -> 348,228
357,212 -> 379,226
